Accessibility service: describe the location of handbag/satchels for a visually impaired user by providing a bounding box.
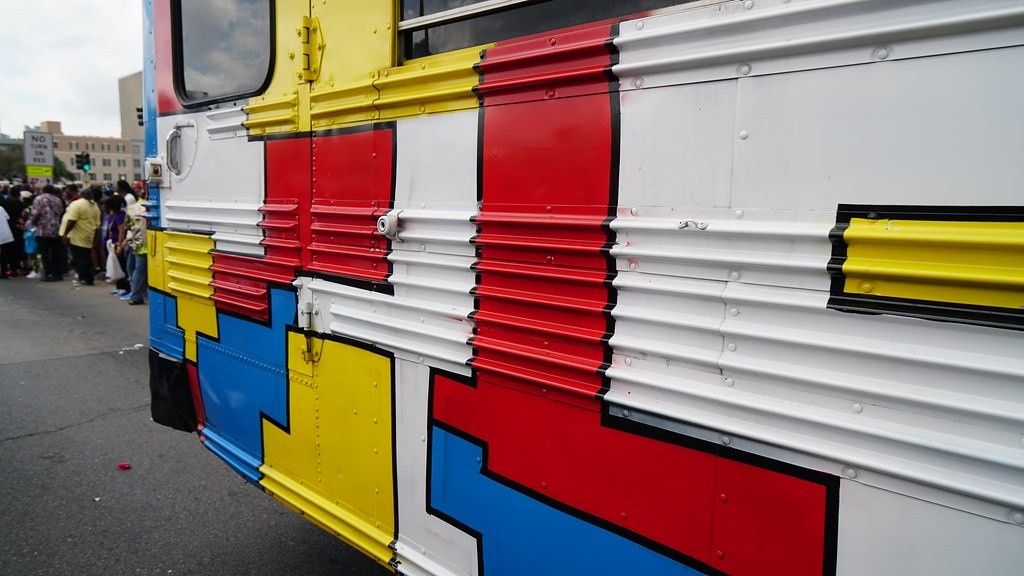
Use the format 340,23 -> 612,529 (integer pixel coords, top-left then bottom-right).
105,238 -> 125,283
24,228 -> 38,253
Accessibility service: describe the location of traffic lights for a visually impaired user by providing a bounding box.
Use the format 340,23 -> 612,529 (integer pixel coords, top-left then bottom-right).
82,151 -> 90,172
76,154 -> 82,169
137,105 -> 144,126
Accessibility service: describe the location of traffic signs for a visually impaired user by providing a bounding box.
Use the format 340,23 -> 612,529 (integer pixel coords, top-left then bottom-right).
25,166 -> 54,178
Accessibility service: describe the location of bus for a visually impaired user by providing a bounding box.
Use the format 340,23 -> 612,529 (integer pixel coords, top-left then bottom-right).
140,0 -> 1022,576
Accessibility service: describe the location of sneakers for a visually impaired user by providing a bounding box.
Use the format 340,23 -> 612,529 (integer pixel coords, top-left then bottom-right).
26,270 -> 41,278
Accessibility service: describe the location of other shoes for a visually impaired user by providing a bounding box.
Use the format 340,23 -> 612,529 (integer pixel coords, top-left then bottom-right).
109,289 -> 119,294
120,291 -> 133,300
128,297 -> 144,305
114,289 -> 131,296
8,275 -> 18,280
72,279 -> 96,286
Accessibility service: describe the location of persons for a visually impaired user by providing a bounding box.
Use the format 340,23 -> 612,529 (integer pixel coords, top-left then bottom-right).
0,175 -> 148,306
